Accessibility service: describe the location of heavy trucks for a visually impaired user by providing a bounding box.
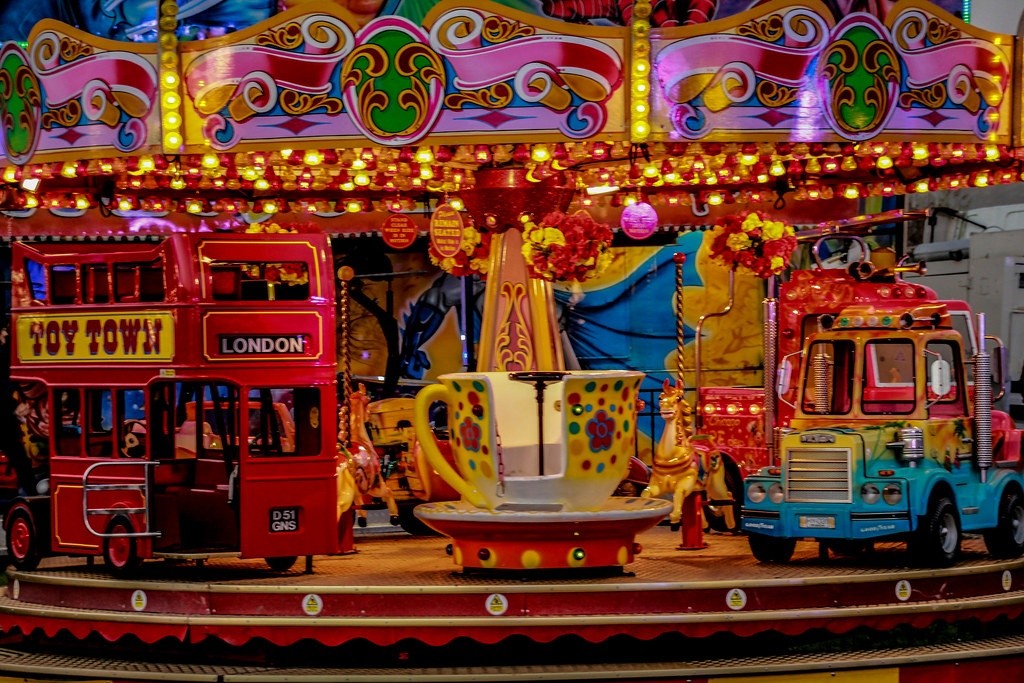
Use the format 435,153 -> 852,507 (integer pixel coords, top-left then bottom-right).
739,301 -> 1023,563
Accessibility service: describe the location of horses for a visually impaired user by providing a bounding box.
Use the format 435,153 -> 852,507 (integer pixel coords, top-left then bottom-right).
336,382 -> 400,527
640,379 -> 738,537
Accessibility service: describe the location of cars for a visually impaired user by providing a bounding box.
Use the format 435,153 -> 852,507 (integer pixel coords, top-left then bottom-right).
170,399 -> 297,453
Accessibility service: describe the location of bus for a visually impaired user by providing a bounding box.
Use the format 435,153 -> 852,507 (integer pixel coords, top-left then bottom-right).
3,239 -> 361,572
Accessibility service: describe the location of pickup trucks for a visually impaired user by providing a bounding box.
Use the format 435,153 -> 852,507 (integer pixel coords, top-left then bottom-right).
690,267 -> 1023,527
